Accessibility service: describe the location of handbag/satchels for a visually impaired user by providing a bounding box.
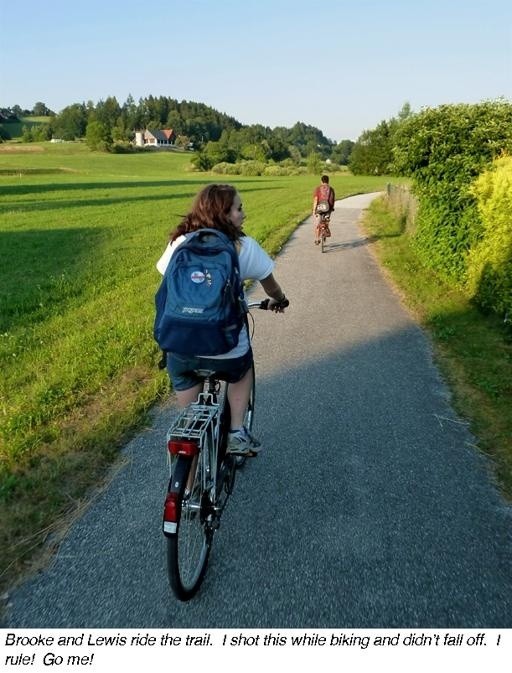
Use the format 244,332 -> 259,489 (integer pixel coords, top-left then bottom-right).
315,200 -> 330,214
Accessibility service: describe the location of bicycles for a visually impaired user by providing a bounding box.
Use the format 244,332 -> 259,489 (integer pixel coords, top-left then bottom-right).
312,209 -> 334,253
162,298 -> 289,602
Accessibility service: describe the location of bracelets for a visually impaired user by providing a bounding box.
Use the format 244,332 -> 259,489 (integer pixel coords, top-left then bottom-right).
280,296 -> 285,301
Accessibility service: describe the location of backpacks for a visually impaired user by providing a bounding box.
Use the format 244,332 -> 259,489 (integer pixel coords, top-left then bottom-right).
154,226 -> 249,369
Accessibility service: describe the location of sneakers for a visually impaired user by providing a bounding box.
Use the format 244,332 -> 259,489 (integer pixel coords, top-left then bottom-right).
225,425 -> 263,456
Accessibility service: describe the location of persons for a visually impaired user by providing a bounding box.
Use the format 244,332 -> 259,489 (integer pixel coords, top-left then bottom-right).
154,184 -> 287,499
313,175 -> 335,245
